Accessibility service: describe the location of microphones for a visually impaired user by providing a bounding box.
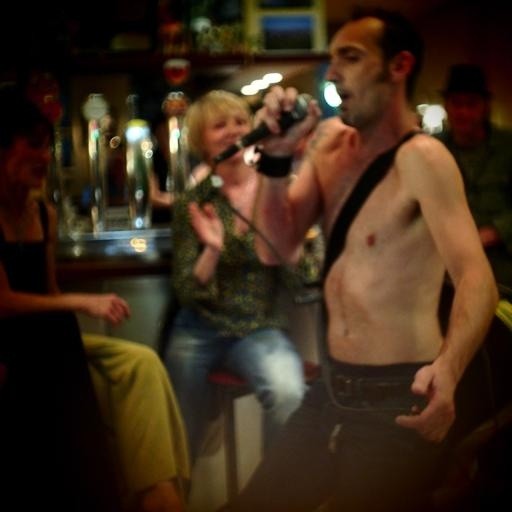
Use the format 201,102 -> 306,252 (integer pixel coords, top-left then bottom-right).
212,93 -> 313,163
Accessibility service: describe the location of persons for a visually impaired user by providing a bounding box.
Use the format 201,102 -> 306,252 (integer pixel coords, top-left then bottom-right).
140,117 -> 212,222
430,64 -> 512,508
1,95 -> 189,511
214,7 -> 498,511
161,89 -> 307,500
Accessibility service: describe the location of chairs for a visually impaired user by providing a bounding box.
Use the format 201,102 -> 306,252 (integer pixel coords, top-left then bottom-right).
1,307 -> 136,511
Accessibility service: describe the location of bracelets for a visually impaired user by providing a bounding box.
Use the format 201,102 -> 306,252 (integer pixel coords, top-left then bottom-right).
255,154 -> 292,177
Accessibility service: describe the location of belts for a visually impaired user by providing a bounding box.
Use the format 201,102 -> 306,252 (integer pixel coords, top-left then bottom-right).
321,375 -> 412,419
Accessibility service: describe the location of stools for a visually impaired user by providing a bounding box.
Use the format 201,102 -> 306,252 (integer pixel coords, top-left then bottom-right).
206,359 -> 322,505
